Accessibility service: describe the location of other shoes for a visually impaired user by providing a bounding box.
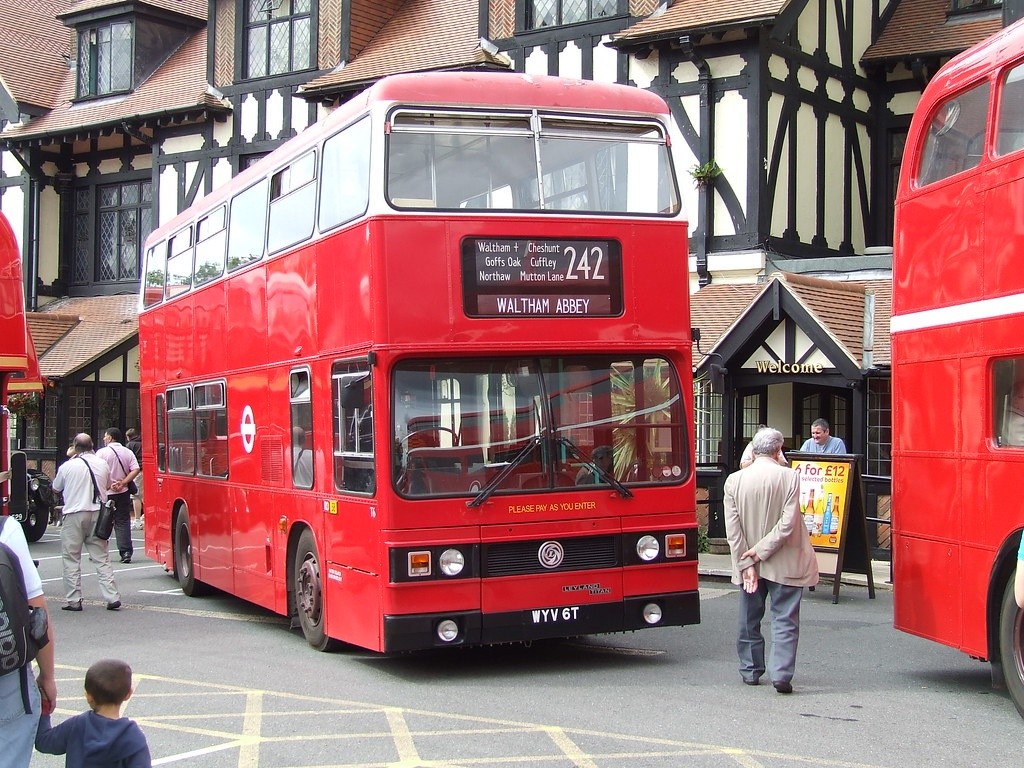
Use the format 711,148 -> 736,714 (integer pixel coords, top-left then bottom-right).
773,681 -> 792,693
121,552 -> 131,562
130,514 -> 145,531
107,601 -> 121,610
62,606 -> 82,611
743,678 -> 759,685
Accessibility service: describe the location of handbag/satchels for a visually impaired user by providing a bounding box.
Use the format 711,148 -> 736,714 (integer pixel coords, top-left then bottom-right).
128,481 -> 137,495
95,503 -> 117,540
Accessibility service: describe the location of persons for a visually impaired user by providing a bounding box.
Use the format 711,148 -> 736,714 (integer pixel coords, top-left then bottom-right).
53,432 -> 121,611
0,513 -> 57,768
723,424 -> 820,693
282,425 -> 313,488
577,446 -> 636,484
96,428 -> 144,563
34,660 -> 151,768
799,418 -> 847,453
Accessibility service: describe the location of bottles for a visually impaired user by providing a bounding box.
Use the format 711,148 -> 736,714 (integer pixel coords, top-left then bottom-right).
799,489 -> 839,538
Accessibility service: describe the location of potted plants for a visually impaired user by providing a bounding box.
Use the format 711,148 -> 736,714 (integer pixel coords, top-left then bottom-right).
687,159 -> 724,190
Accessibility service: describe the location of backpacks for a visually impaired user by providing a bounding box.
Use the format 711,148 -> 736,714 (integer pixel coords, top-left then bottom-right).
0,516 -> 49,714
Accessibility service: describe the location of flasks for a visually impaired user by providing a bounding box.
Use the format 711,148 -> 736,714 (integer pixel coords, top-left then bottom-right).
95,500 -> 115,540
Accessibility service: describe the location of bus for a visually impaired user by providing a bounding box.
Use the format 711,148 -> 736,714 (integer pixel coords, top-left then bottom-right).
888,14 -> 1024,717
136,68 -> 731,655
0,215 -> 52,544
